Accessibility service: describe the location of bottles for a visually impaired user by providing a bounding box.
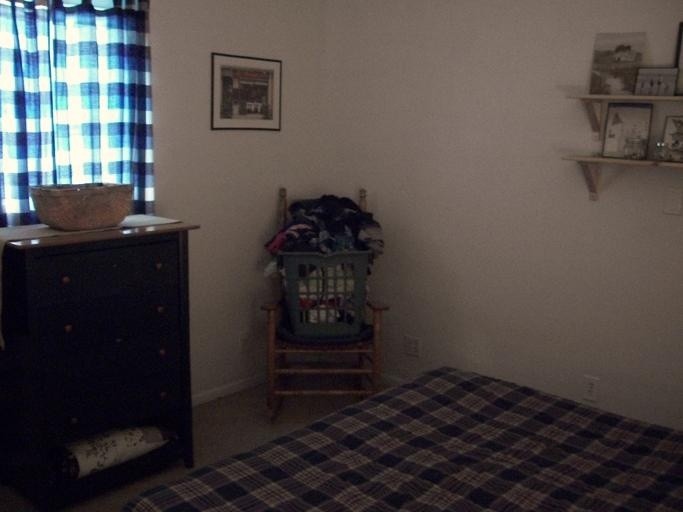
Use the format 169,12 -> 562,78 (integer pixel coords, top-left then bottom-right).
654,142 -> 670,162
622,136 -> 646,160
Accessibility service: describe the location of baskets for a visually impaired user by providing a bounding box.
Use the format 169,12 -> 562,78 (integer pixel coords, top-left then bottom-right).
279,249 -> 372,340
28,182 -> 134,230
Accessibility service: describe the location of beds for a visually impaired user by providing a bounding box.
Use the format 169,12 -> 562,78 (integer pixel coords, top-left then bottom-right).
120,365 -> 683,512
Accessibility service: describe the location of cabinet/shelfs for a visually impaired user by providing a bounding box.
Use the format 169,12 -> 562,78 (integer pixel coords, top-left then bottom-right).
0,213 -> 200,512
560,93 -> 683,201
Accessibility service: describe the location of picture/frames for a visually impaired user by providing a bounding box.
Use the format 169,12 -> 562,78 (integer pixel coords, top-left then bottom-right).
601,103 -> 653,160
211,52 -> 282,131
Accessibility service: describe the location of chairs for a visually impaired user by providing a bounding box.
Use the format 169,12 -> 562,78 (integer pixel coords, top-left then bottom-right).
260,188 -> 390,409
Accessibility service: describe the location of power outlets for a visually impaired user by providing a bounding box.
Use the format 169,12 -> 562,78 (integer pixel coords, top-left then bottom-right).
580,374 -> 600,403
